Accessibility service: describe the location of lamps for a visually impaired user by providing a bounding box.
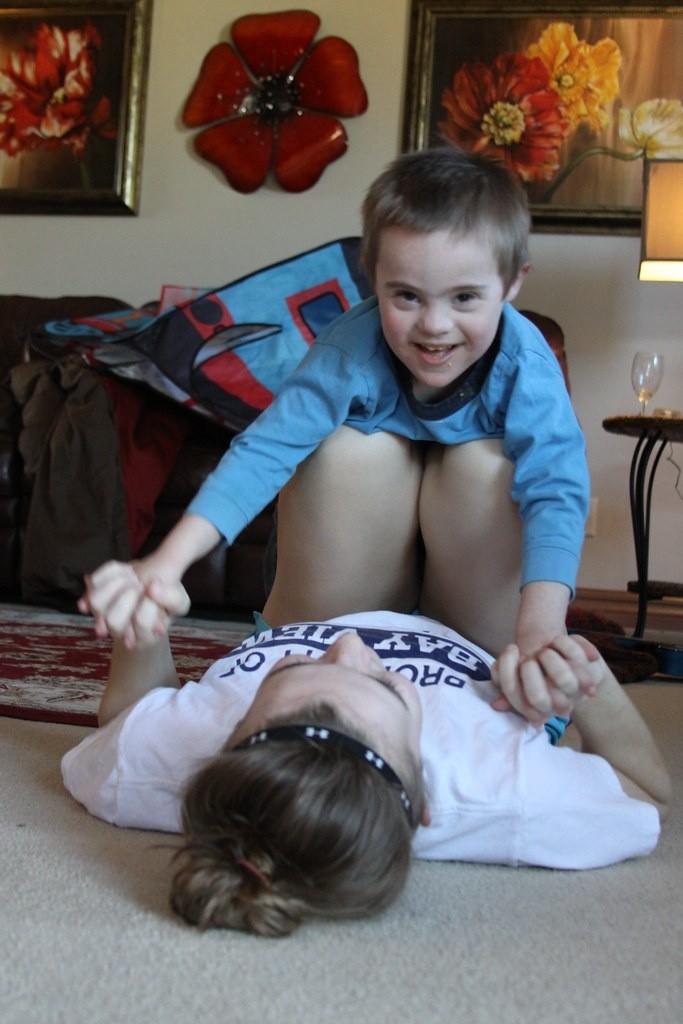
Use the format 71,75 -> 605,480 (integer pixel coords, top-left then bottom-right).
637,158 -> 683,283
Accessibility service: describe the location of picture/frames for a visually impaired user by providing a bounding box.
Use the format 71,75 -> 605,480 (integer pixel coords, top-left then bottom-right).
0,0 -> 154,216
401,0 -> 683,237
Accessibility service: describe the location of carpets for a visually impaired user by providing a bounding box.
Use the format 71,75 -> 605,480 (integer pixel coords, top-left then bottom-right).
0,604 -> 255,728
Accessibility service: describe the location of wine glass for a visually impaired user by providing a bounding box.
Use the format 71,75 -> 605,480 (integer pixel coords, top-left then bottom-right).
631,353 -> 664,415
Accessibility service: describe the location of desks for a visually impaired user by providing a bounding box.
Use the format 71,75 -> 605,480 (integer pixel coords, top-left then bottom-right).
602,416 -> 683,638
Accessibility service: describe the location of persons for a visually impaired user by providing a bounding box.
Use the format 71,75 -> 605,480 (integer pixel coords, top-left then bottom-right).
89,145 -> 589,725
61,425 -> 672,937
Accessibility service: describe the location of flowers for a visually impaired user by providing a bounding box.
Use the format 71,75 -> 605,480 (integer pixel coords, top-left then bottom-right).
182,9 -> 369,194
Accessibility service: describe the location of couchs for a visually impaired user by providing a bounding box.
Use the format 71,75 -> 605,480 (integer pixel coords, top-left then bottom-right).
0,296 -> 568,623
